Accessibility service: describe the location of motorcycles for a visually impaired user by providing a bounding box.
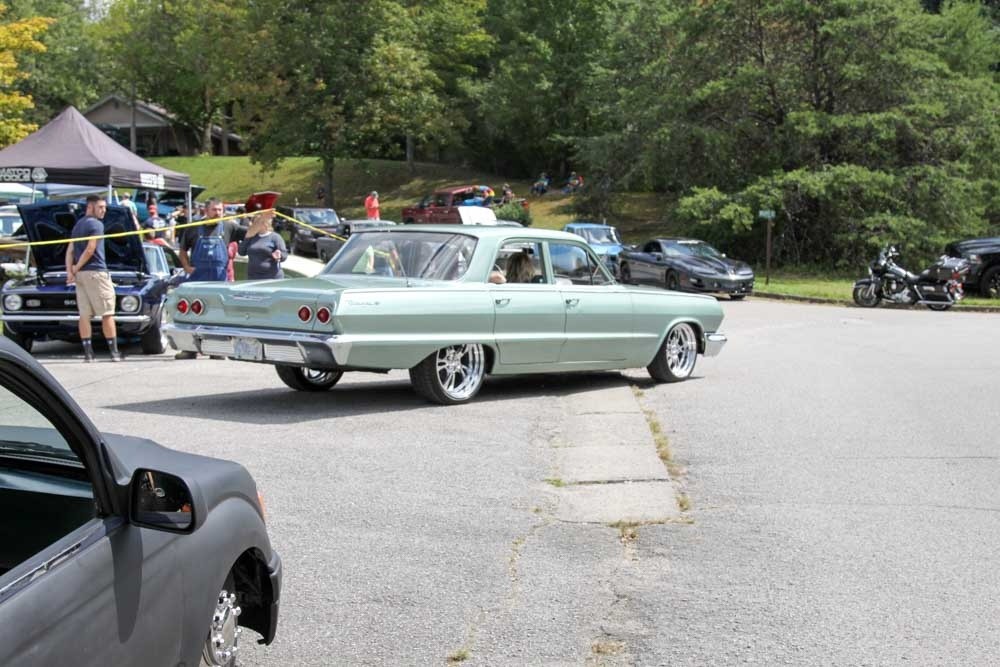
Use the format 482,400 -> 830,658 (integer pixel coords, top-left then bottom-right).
853,241 -> 970,311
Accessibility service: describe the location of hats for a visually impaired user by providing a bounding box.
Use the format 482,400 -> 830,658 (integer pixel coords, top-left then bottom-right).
372,192 -> 378,199
149,205 -> 157,213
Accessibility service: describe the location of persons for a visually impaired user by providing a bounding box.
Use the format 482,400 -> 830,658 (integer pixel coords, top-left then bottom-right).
118,191 -> 299,359
365,190 -> 380,220
65,194 -> 130,363
471,172 -> 584,206
488,251 -> 535,284
352,239 -> 400,276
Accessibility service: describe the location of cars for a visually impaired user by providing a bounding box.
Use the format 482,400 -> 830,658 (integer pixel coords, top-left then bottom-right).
0,199 -> 191,354
617,235 -> 755,300
156,223 -> 729,406
0,182 -> 45,205
942,237 -> 1000,300
202,204 -> 539,281
0,333 -> 283,667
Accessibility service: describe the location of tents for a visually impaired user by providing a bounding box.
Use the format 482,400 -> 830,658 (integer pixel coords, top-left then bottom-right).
0,105 -> 193,272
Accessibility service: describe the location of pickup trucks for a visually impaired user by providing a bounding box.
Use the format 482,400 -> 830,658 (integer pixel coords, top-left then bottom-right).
533,222 -> 627,276
401,185 -> 529,227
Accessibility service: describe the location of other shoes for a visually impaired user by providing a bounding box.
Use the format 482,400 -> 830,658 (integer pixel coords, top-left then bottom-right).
85,352 -> 96,363
210,355 -> 225,360
176,351 -> 197,359
111,352 -> 125,361
